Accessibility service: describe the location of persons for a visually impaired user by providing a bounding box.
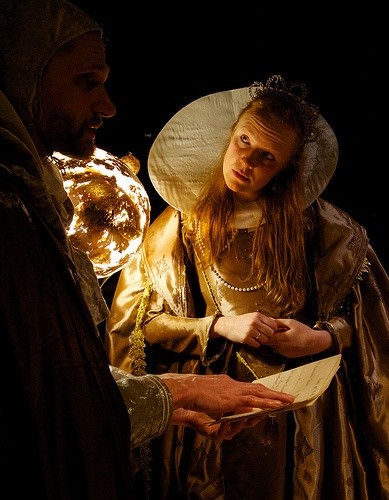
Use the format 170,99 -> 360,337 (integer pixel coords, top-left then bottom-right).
102,74 -> 389,499
0,0 -> 294,500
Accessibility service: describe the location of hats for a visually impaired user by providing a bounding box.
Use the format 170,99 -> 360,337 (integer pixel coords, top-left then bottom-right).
0,0 -> 103,128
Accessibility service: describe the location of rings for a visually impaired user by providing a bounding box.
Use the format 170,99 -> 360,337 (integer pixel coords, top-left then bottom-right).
256,333 -> 262,340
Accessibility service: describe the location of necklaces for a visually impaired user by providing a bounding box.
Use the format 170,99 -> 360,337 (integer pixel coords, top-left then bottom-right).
198,225 -> 277,292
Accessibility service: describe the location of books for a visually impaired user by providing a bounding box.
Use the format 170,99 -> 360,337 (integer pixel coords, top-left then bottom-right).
205,354 -> 341,426
